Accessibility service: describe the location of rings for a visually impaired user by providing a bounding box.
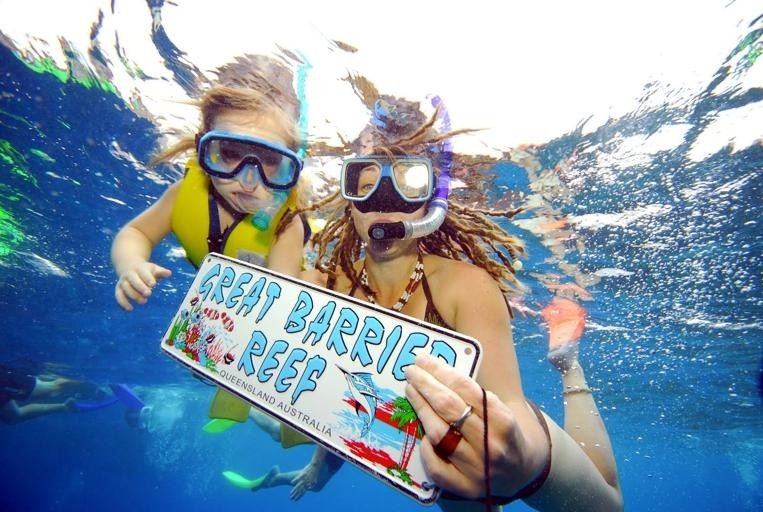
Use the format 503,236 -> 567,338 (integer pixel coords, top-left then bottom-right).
299,93 -> 624,512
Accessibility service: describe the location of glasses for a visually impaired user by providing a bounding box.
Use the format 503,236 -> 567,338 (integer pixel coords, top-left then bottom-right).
192,131 -> 304,191
338,155 -> 433,214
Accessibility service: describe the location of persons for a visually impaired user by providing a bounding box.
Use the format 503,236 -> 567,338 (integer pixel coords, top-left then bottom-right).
450,405 -> 473,427
108,53 -> 326,449
204,407 -> 346,503
0,364 -> 84,425
